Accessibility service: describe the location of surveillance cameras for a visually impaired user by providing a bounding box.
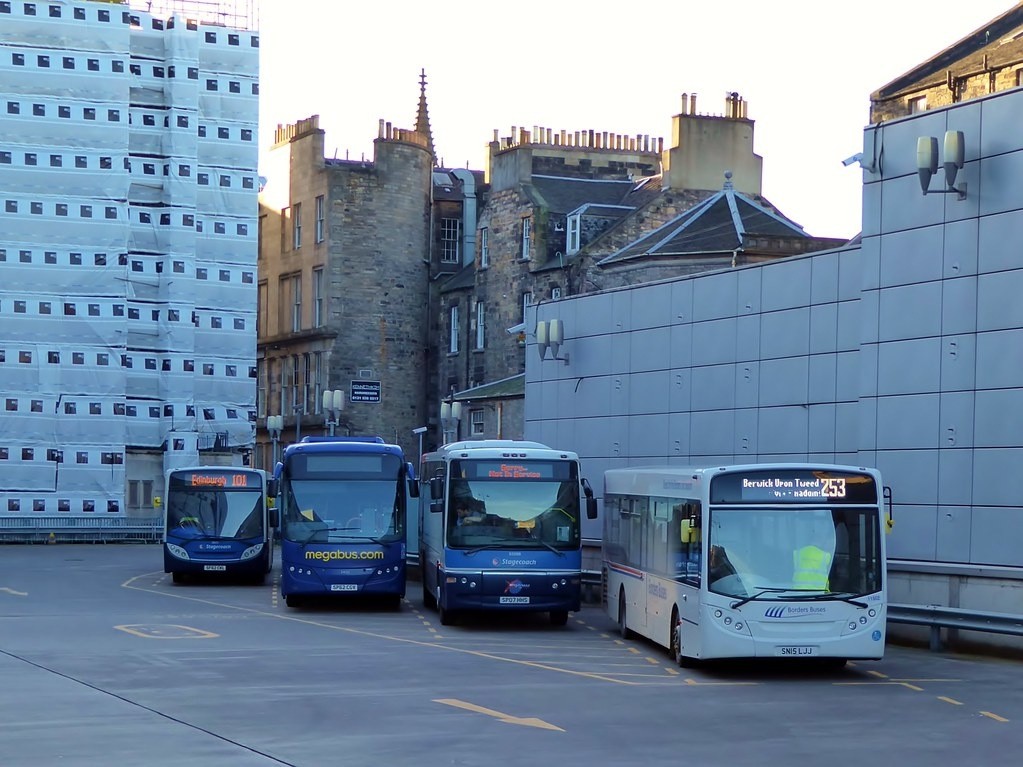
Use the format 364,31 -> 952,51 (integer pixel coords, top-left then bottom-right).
842,152 -> 863,167
412,427 -> 427,434
506,323 -> 526,335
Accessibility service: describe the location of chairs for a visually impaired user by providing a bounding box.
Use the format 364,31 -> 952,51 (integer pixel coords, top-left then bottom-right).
298,492 -> 328,521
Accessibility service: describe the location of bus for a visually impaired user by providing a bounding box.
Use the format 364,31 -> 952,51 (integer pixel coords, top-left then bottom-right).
153,465 -> 274,583
600,462 -> 893,673
270,435 -> 422,613
416,438 -> 598,630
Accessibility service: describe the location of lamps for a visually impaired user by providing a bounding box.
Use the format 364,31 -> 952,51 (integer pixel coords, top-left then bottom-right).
916,129 -> 968,201
537,320 -> 570,366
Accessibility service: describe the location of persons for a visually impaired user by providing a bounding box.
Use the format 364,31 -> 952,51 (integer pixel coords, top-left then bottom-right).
454,503 -> 487,529
790,543 -> 834,593
